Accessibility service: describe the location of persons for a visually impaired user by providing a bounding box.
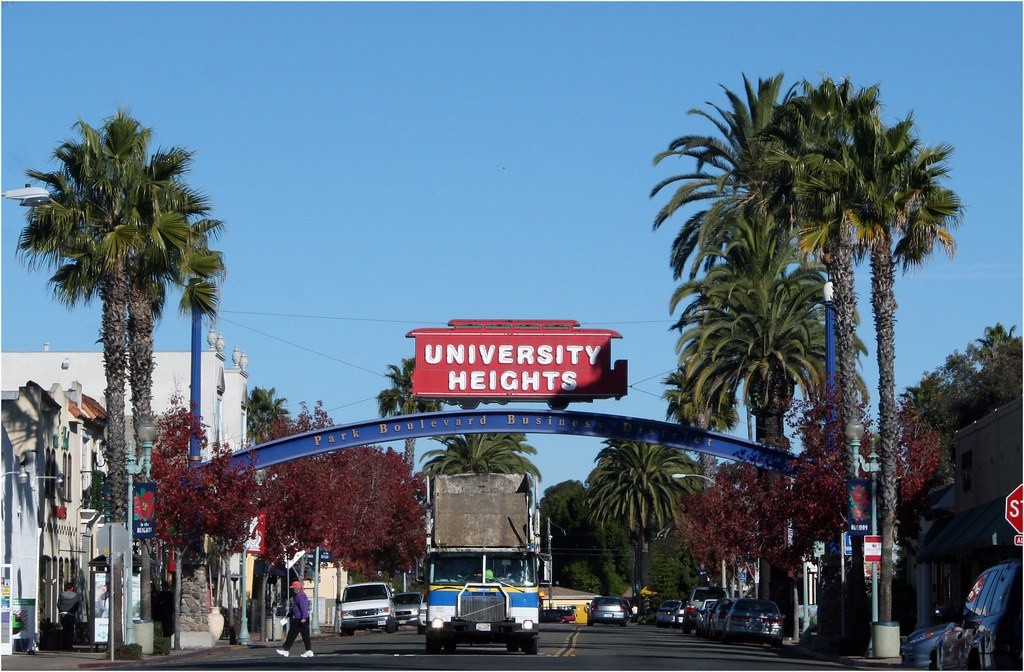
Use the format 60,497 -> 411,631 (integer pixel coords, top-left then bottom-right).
57,582 -> 80,647
277,581 -> 314,657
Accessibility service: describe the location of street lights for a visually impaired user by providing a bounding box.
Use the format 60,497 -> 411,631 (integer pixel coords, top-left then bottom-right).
844,416 -> 881,658
671,472 -> 727,591
124,415 -> 156,644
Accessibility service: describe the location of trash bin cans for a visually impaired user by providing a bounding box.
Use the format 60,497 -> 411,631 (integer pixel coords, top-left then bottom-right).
872,622 -> 901,658
265,616 -> 284,640
132,620 -> 155,655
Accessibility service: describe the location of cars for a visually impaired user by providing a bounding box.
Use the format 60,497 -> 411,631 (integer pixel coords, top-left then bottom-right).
708,599 -> 738,640
675,599 -> 688,628
548,609 -> 576,623
694,598 -> 719,638
903,609 -> 1023,671
586,596 -> 631,627
721,598 -> 787,649
656,599 -> 685,627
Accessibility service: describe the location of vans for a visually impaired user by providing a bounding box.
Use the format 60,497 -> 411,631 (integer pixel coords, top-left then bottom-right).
335,582 -> 397,636
961,558 -> 1023,613
682,588 -> 728,635
394,592 -> 425,628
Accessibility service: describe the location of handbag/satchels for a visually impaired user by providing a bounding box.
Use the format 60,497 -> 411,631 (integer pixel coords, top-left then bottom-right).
307,600 -> 312,610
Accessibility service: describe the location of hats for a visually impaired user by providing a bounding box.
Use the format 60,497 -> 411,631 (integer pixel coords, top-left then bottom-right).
288,581 -> 301,588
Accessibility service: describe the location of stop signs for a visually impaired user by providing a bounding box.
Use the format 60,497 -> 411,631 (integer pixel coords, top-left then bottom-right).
1006,484 -> 1024,534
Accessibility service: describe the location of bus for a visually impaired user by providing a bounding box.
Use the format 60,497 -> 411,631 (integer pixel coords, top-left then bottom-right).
425,470 -> 554,655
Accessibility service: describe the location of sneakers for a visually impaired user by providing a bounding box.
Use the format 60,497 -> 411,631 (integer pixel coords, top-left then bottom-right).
301,652 -> 314,658
277,649 -> 289,657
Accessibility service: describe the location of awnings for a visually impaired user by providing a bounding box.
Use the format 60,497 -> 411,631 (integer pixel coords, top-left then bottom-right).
917,494 -> 1019,561
